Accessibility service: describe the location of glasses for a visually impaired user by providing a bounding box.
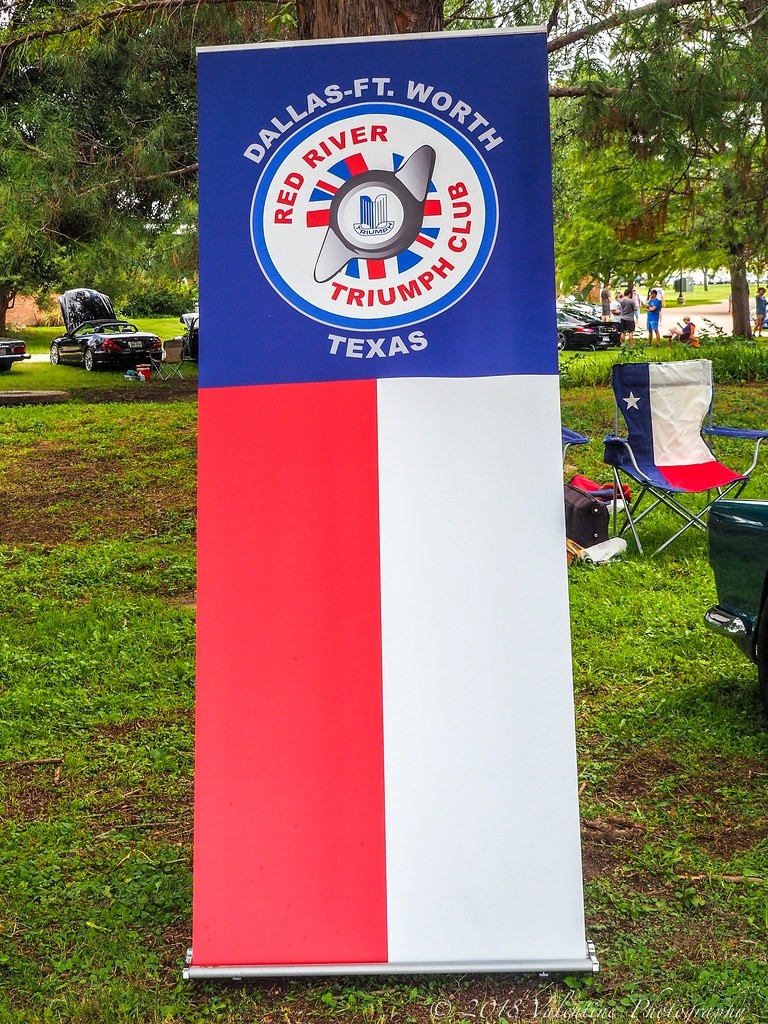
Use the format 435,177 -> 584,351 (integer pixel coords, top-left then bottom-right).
683,318 -> 686,322
632,289 -> 635,292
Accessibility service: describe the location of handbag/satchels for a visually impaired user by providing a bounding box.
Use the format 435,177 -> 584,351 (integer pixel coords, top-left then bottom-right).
564,484 -> 610,549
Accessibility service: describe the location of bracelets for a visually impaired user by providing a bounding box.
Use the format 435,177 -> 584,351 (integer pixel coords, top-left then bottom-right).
647,306 -> 649,309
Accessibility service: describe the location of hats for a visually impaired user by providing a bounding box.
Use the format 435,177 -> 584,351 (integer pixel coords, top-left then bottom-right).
653,280 -> 661,287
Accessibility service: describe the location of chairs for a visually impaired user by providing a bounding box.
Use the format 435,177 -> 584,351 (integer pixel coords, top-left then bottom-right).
151,339 -> 185,381
602,359 -> 768,559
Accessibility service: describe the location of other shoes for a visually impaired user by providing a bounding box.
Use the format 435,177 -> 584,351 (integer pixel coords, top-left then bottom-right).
642,344 -> 652,348
753,334 -> 756,338
663,334 -> 672,338
636,320 -> 638,324
656,344 -> 661,348
759,335 -> 762,337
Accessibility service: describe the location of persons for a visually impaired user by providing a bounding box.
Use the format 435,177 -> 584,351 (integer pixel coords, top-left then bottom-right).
602,284 -> 611,322
728,293 -> 732,312
663,316 -> 695,349
649,281 -> 665,326
632,288 -> 641,323
617,289 -> 638,349
752,287 -> 768,337
610,292 -> 622,322
643,290 -> 661,348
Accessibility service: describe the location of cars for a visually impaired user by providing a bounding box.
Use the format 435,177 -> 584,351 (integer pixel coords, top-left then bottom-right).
49,287 -> 162,372
0,336 -> 31,371
557,303 -> 620,351
